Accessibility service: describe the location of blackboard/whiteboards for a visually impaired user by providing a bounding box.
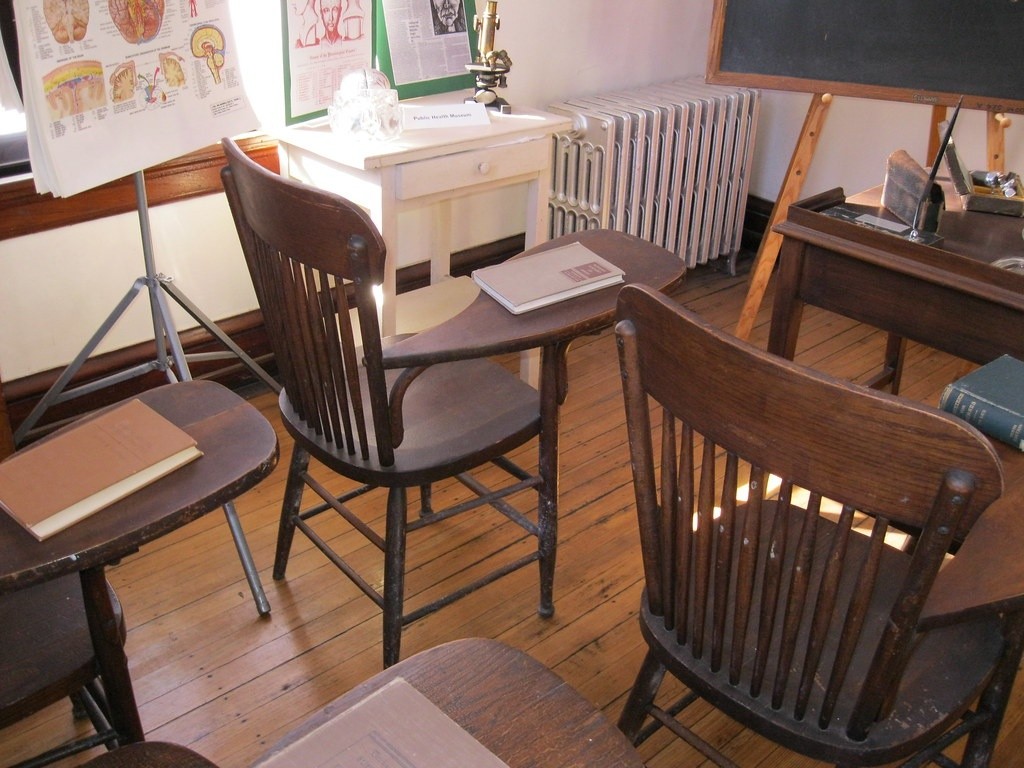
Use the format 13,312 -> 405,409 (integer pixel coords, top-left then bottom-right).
704,0 -> 1024,115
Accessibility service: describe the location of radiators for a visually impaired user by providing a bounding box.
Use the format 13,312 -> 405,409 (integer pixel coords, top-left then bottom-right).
547,74 -> 760,275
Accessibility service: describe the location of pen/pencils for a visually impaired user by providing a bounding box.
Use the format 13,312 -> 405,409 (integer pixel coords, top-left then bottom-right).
912,94 -> 964,231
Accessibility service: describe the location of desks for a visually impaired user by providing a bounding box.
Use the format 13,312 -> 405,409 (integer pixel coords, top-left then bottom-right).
274,91 -> 572,391
765,167 -> 1024,396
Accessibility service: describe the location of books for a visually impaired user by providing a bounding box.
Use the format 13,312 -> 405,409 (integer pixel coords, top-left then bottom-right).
249,676 -> 513,768
0,398 -> 206,542
472,240 -> 627,315
940,352 -> 1023,452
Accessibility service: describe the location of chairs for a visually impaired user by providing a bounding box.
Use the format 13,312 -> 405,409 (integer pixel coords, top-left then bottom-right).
615,284 -> 1024,768
0,380 -> 279,768
220,139 -> 686,671
73,638 -> 644,768
